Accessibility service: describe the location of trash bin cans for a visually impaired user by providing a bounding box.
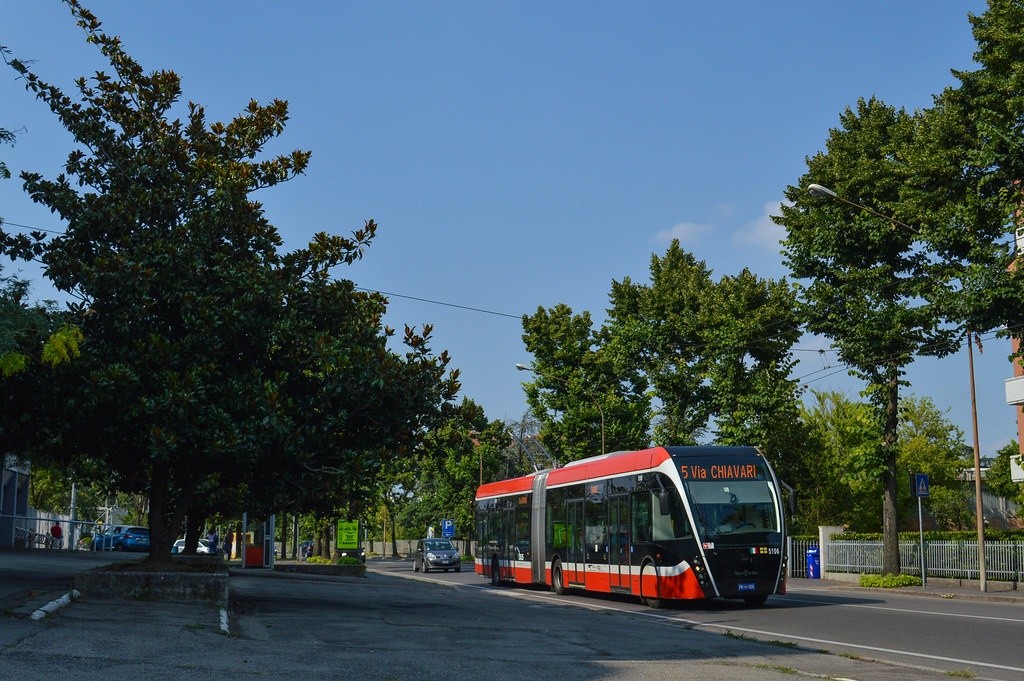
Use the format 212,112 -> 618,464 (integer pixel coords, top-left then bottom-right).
245,545 -> 263,568
806,548 -> 820,579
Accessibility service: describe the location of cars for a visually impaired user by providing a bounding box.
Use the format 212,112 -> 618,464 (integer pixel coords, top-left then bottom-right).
336,543 -> 366,564
514,541 -> 531,553
173,539 -> 225,558
90,525 -> 150,553
412,538 -> 461,572
483,540 -> 502,552
298,540 -> 322,557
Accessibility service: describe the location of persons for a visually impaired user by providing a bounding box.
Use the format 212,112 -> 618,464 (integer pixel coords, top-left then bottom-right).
206,525 -> 219,545
589,517 -> 603,544
725,508 -> 749,531
222,529 -> 233,561
50,521 -> 62,549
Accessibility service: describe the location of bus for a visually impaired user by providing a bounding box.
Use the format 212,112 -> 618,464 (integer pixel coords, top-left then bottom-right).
473,446 -> 796,608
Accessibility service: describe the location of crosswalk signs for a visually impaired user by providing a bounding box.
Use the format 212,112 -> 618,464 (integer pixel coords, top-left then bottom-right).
915,473 -> 929,497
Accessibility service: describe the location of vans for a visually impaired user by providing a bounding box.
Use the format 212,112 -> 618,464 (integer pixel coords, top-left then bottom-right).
221,532 -> 255,559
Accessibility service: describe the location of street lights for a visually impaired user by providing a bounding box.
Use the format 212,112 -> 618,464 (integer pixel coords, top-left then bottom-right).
516,363 -> 605,454
807,184 -> 989,593
